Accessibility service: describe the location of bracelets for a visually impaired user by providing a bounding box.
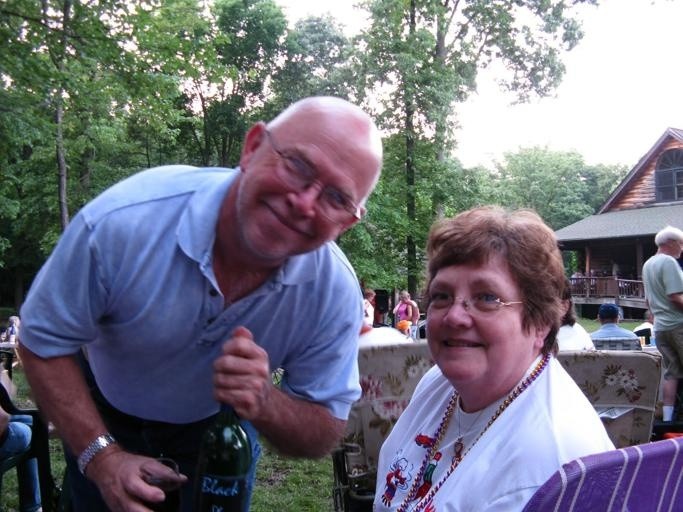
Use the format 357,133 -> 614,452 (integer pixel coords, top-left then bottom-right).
77,432 -> 118,475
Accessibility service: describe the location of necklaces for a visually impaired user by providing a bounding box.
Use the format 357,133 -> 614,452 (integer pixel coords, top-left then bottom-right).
397,352 -> 551,512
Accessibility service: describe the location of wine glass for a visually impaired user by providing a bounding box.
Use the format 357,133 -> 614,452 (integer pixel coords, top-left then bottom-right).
1,331 -> 6,344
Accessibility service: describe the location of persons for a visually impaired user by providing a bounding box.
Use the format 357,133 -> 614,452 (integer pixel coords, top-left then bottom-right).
372,205 -> 616,512
362,287 -> 420,340
16,95 -> 383,512
555,226 -> 682,422
1,317 -> 43,512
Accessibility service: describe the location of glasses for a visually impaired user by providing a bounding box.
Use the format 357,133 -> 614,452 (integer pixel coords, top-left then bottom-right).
264,123 -> 360,224
418,289 -> 526,311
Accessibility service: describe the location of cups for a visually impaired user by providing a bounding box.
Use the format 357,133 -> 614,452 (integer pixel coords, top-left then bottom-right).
407,325 -> 418,343
639,336 -> 645,347
139,456 -> 183,511
649,336 -> 656,346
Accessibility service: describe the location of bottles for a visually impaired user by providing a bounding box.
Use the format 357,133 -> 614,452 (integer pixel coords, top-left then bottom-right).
191,398 -> 252,512
8,325 -> 15,343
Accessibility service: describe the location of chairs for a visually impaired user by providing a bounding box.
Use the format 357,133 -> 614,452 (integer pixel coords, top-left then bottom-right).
0,349 -> 62,511
329,327 -> 683,512
